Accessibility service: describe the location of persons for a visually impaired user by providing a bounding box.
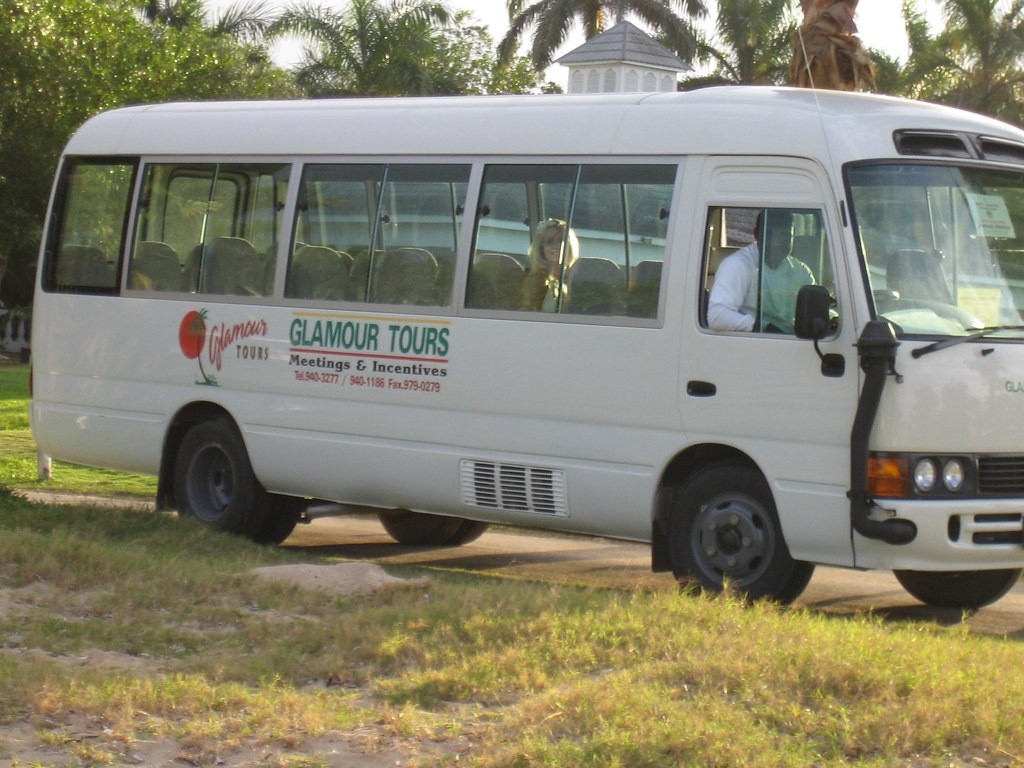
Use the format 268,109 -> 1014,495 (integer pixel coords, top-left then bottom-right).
707,208 -> 838,335
520,218 -> 580,313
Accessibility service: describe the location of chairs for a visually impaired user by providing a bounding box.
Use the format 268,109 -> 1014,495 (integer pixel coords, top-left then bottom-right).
887,248 -> 953,306
705,247 -> 741,291
54,237 -> 663,318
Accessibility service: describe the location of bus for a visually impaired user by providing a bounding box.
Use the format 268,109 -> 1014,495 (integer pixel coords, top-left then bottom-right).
31,85 -> 1024,613
